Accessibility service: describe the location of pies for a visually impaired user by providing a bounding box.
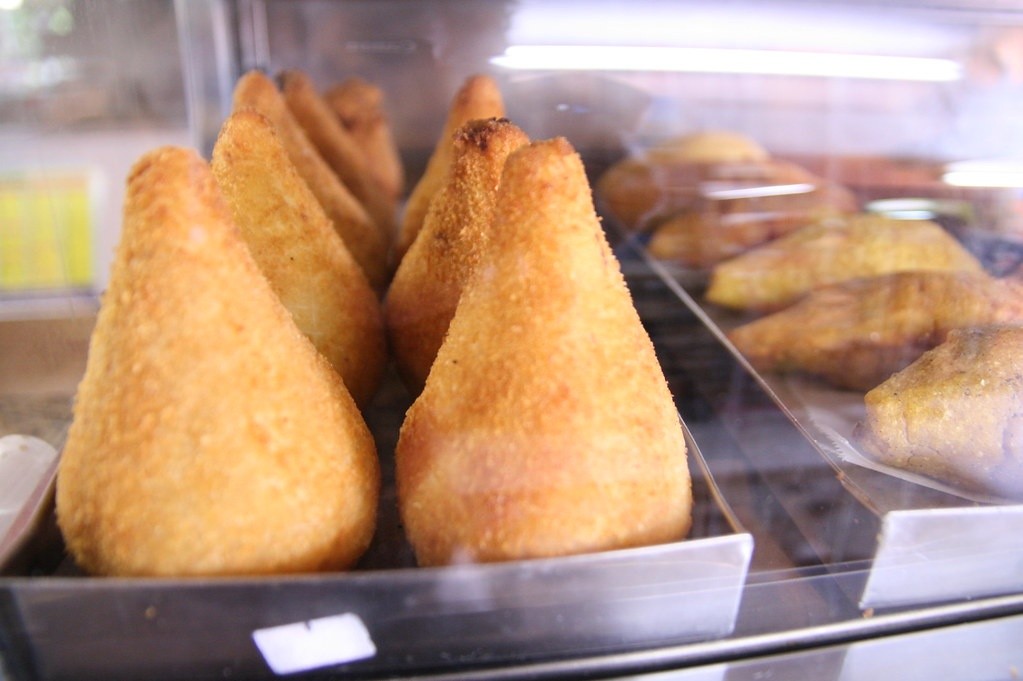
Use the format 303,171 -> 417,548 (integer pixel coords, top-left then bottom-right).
594,123 -> 1022,504
